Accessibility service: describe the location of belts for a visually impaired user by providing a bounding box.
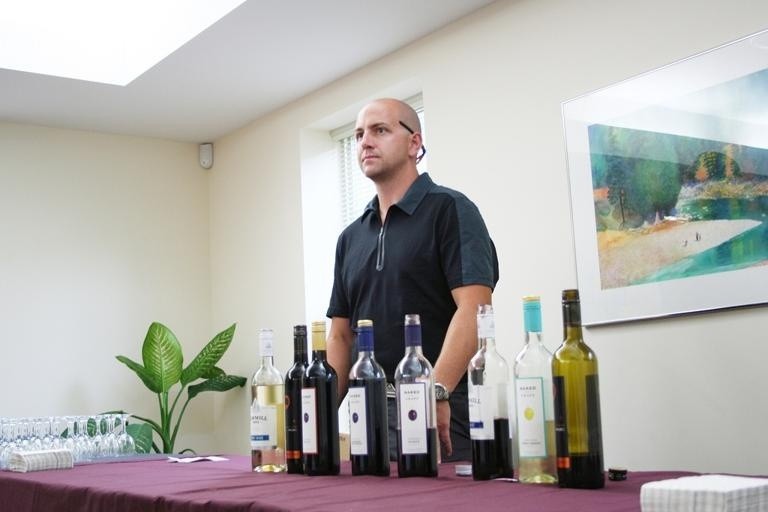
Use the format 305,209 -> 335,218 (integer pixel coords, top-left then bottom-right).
382,380 -> 469,400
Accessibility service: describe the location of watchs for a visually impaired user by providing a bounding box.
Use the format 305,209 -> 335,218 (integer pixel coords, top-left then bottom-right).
434,383 -> 452,402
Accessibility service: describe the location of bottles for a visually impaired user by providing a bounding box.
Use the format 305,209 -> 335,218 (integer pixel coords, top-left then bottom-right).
285,325 -> 309,474
467,303 -> 514,482
347,320 -> 391,478
513,297 -> 556,483
552,289 -> 605,491
251,330 -> 288,473
301,322 -> 341,477
394,315 -> 438,478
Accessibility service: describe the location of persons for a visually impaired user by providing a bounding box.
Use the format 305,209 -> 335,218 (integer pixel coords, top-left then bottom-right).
325,99 -> 500,466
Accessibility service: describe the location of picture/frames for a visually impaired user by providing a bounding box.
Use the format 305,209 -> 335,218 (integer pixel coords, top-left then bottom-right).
561,29 -> 768,327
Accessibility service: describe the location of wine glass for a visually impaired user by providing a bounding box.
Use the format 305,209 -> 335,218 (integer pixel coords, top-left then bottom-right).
0,414 -> 136,467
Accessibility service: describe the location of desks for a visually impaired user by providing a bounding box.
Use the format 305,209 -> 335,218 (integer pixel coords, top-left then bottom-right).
0,454 -> 768,511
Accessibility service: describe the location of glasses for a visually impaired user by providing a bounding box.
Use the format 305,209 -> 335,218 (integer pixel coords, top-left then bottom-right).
398,119 -> 427,166
